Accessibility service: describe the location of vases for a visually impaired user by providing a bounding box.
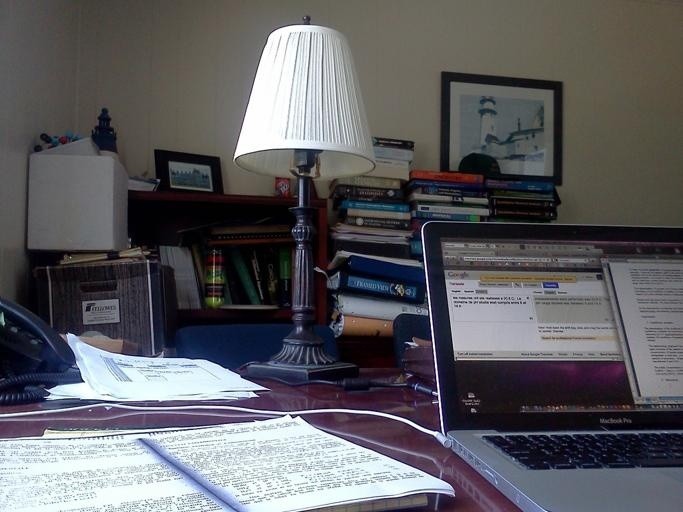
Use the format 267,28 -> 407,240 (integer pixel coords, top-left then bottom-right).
154,148 -> 224,195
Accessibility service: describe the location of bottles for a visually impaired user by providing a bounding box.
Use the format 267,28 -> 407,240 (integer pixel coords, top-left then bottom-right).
203,248 -> 226,308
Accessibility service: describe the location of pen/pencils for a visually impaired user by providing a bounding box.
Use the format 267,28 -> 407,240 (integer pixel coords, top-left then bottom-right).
408,382 -> 438,397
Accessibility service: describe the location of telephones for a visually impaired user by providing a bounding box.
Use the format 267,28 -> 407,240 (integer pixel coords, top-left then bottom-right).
0,296 -> 84,389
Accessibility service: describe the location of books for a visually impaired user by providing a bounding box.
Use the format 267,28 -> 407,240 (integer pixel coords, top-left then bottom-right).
320,136 -> 563,338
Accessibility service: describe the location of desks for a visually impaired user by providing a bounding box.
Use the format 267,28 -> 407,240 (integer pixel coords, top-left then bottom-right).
0,367 -> 523,512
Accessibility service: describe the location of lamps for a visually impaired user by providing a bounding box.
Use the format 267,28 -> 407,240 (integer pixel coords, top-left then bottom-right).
234,15 -> 377,386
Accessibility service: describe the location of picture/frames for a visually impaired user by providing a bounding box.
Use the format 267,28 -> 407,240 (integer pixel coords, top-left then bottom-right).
440,70 -> 563,186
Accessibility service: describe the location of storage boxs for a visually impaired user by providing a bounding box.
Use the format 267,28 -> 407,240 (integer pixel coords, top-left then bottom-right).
32,261 -> 163,357
28,154 -> 129,251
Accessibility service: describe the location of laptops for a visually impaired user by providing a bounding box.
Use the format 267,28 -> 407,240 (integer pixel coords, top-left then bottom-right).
421,219 -> 683,512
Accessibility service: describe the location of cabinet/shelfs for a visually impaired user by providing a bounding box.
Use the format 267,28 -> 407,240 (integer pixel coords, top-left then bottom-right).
127,190 -> 329,326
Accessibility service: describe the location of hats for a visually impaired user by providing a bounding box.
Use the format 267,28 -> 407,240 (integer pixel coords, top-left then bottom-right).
458,153 -> 520,181
